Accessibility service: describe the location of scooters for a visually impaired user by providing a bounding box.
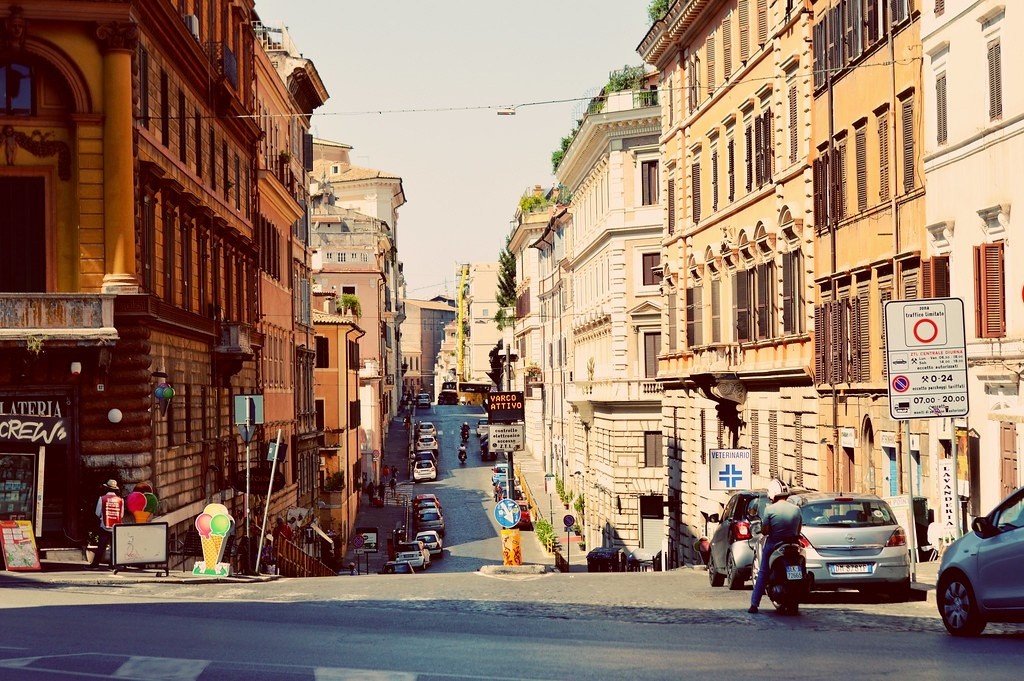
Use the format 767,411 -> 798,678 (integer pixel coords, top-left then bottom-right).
456,444 -> 469,462
460,422 -> 470,441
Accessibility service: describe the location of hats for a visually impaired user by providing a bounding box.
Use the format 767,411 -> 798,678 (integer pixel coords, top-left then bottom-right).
102,479 -> 119,490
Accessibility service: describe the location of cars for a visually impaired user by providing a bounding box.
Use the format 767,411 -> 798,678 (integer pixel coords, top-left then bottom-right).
706,492 -> 771,590
417,393 -> 431,408
415,435 -> 438,451
936,486 -> 1024,640
415,530 -> 443,555
410,494 -> 445,536
382,560 -> 415,574
797,491 -> 912,597
411,451 -> 437,466
476,418 -> 499,461
416,420 -> 437,438
412,460 -> 437,480
491,463 -> 531,528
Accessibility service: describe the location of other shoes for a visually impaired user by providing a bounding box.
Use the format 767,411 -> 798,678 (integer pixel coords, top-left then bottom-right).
748,600 -> 760,613
84,564 -> 100,570
106,565 -> 115,571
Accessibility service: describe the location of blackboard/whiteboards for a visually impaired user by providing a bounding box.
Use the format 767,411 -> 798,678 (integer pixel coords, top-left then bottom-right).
114,522 -> 168,565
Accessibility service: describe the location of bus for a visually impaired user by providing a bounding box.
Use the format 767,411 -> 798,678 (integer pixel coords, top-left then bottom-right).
438,381 -> 457,406
458,382 -> 491,406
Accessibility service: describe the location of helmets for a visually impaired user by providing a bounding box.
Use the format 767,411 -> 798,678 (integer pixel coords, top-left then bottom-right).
767,479 -> 791,502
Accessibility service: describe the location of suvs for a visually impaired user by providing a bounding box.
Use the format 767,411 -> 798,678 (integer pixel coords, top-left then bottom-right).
394,540 -> 432,570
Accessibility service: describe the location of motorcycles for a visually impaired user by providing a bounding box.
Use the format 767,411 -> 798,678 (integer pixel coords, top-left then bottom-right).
749,507 -> 814,615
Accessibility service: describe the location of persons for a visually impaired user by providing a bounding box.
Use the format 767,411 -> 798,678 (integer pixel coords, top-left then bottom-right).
84,479 -> 124,571
400,385 -> 415,429
237,517 -> 341,577
457,443 -> 467,459
389,477 -> 396,498
461,421 -> 470,438
389,465 -> 396,478
747,479 -> 802,613
348,562 -> 358,576
367,482 -> 374,504
383,465 -> 389,483
377,482 -> 385,501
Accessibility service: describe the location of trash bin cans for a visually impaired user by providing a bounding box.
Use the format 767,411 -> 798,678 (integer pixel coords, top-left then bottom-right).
587,547 -> 619,573
914,497 -> 928,546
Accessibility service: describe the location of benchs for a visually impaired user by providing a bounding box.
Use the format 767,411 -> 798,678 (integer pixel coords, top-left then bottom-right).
167,532 -> 237,572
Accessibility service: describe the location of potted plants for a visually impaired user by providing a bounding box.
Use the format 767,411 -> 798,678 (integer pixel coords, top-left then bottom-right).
280,151 -> 291,163
576,541 -> 586,551
564,496 -> 570,510
526,362 -> 542,382
336,293 -> 362,319
574,525 -> 581,535
83,532 -> 110,563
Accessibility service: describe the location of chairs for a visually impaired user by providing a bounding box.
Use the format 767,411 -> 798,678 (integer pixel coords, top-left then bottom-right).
828,509 -> 864,524
